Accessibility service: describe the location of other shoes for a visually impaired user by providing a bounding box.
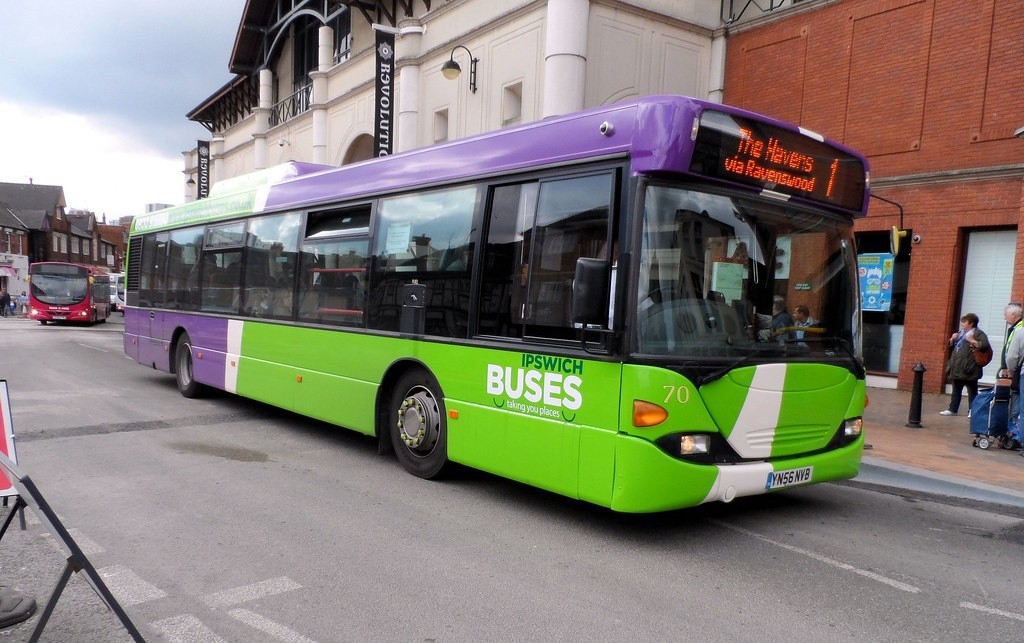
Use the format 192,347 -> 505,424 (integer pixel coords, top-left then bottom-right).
968,409 -> 972,418
939,409 -> 957,415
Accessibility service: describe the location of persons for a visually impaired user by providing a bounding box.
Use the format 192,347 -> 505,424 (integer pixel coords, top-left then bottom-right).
0,288 -> 29,318
793,304 -> 820,347
1000,304 -> 1024,457
573,234 -> 654,329
345,235 -> 534,339
186,254 -> 239,310
940,313 -> 991,418
772,295 -> 798,345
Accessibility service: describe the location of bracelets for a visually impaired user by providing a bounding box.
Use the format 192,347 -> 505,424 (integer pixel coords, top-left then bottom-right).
972,341 -> 977,345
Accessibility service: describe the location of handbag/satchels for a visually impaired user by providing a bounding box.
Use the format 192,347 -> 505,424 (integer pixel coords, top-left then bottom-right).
971,333 -> 993,367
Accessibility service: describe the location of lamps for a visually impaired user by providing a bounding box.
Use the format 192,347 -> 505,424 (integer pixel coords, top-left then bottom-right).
441,44 -> 480,93
186,170 -> 198,187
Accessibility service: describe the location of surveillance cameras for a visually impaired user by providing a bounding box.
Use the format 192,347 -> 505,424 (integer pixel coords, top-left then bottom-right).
279,139 -> 285,146
913,236 -> 920,244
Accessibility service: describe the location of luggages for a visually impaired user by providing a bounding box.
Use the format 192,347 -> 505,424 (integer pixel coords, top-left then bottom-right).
970,367 -> 1014,450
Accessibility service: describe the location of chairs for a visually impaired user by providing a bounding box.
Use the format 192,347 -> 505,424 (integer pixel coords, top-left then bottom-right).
173,259 -> 356,314
536,280 -> 571,322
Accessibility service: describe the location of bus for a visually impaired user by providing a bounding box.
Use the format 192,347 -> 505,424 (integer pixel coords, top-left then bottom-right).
25,262 -> 111,327
108,272 -> 125,312
124,96 -> 907,515
116,276 -> 125,315
